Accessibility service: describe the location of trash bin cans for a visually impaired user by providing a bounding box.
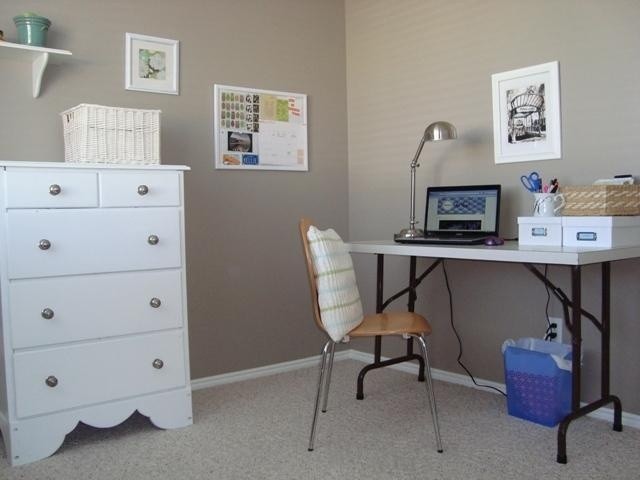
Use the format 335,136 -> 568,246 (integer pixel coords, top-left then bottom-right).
501,337 -> 572,427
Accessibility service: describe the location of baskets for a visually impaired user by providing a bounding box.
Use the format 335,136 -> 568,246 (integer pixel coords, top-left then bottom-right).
58,102 -> 163,167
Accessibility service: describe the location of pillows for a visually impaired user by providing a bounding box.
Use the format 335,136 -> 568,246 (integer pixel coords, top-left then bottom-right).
305,224 -> 365,344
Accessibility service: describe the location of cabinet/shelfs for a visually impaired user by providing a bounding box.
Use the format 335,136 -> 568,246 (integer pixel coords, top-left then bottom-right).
0,161 -> 191,468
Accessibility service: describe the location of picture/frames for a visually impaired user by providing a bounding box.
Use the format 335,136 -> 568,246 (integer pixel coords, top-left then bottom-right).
212,84 -> 310,173
124,31 -> 181,97
491,60 -> 563,165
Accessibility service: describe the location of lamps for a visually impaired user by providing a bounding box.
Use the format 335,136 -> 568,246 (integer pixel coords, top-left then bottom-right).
393,120 -> 457,242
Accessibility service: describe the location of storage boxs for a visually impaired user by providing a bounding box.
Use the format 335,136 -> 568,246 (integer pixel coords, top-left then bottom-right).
515,215 -> 639,249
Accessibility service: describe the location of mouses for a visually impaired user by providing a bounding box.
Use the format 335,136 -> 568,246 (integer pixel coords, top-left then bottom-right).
485,235 -> 504,245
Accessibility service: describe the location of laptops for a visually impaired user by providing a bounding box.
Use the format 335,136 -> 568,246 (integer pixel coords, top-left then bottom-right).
395,185 -> 501,245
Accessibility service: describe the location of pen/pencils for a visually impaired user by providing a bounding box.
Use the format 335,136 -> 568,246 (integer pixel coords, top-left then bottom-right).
539,178 -> 559,193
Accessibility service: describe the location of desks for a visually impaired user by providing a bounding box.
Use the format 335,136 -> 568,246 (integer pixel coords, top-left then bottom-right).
343,236 -> 639,465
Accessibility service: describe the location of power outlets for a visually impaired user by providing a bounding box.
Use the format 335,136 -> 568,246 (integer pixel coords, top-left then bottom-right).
546,316 -> 563,344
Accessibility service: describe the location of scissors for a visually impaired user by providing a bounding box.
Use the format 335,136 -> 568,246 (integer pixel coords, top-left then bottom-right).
521,172 -> 540,192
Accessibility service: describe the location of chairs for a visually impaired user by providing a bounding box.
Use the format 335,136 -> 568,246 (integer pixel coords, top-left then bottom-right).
298,219 -> 444,456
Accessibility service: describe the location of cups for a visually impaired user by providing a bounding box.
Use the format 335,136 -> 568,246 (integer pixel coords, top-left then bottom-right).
534,192 -> 566,217
